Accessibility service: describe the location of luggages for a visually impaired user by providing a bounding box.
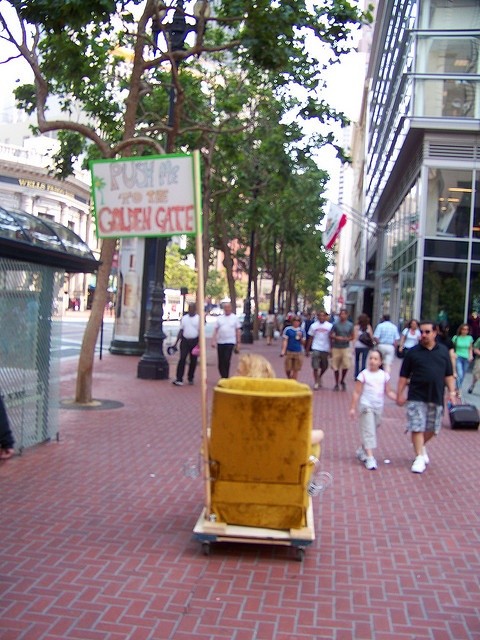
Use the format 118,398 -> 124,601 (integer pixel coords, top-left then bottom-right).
449,377 -> 480,429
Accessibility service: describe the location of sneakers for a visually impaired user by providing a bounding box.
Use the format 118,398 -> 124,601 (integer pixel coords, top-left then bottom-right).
411,456 -> 426,473
355,447 -> 367,462
364,456 -> 377,470
314,383 -> 318,389
173,380 -> 183,385
335,386 -> 339,390
423,446 -> 429,464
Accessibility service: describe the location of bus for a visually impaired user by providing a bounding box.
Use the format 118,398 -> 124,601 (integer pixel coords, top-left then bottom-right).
161,288 -> 184,321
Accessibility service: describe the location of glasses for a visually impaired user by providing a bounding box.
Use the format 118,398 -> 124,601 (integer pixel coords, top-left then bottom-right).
420,330 -> 430,334
293,319 -> 300,322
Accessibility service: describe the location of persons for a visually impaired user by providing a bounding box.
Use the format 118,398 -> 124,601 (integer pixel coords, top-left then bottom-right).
467,335 -> 480,394
349,350 -> 397,470
211,302 -> 242,379
277,307 -> 305,335
397,322 -> 457,473
373,313 -> 400,378
279,317 -> 306,380
352,313 -> 375,381
436,322 -> 458,378
399,319 -> 422,359
305,311 -> 333,390
329,309 -> 355,391
265,308 -> 276,345
305,310 -> 335,323
451,323 -> 474,398
172,302 -> 203,386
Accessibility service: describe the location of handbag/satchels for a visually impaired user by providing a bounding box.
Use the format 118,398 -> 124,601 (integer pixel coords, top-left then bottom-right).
191,345 -> 200,355
396,346 -> 405,358
359,331 -> 374,348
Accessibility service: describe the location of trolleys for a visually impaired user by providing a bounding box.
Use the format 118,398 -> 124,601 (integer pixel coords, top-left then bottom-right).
191,496 -> 316,561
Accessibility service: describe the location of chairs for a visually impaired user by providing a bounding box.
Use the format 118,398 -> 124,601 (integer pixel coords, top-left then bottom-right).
207,376 -> 321,530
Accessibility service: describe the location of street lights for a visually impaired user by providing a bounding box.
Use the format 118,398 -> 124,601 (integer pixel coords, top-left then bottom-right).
240,165 -> 264,344
137,0 -> 211,378
